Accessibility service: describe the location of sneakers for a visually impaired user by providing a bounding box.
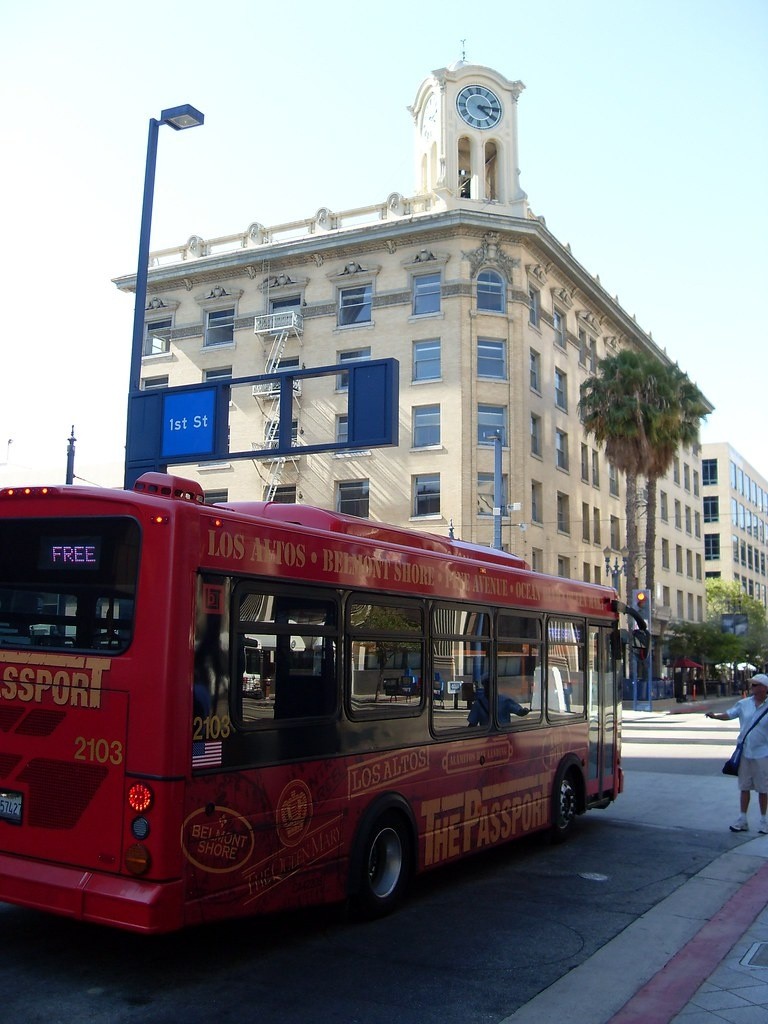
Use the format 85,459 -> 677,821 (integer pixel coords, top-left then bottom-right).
729,818 -> 749,831
758,818 -> 768,834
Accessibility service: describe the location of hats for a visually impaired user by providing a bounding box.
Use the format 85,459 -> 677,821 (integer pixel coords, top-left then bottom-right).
481,672 -> 489,684
747,673 -> 768,686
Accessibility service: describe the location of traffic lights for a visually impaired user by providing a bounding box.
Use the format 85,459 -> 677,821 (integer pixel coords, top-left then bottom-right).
630,587 -> 650,618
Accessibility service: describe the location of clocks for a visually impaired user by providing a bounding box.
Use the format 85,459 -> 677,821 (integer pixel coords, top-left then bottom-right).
456,85 -> 503,130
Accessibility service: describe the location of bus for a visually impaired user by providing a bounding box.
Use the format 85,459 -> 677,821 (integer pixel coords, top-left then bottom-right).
0,469 -> 651,937
241,618 -> 307,699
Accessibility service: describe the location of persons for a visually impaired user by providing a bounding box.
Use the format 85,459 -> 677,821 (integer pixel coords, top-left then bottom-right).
720,670 -> 728,696
467,673 -> 530,728
705,674 -> 768,834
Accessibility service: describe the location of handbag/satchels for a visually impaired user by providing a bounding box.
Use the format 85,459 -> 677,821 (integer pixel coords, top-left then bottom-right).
722,744 -> 743,775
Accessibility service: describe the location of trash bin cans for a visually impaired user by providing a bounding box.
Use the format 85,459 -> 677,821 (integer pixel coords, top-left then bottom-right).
674,679 -> 688,703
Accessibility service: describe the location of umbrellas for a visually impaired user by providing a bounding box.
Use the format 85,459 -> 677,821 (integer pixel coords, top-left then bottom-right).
665,655 -> 704,668
715,662 -> 756,671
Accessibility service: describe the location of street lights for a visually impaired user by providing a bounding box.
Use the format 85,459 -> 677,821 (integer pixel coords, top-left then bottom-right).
122,103 -> 205,492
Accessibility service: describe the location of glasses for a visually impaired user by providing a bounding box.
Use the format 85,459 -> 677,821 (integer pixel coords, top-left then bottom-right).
752,683 -> 763,689
